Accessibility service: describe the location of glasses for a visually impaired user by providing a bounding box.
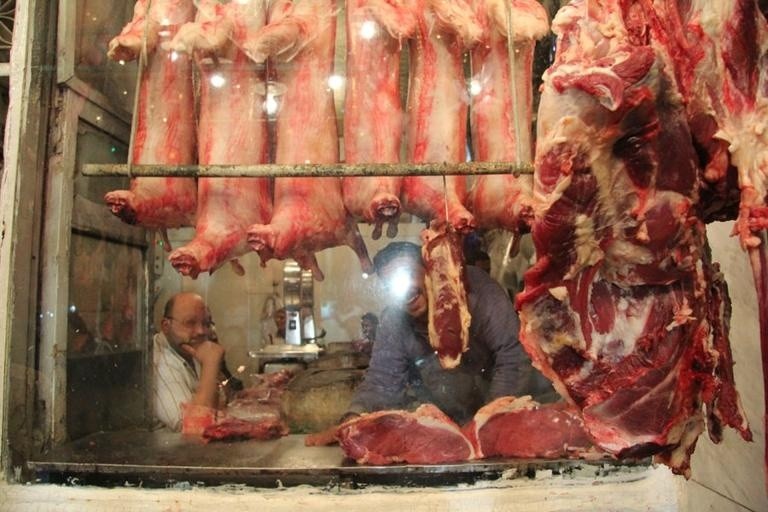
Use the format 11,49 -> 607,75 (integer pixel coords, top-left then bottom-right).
166,317 -> 216,330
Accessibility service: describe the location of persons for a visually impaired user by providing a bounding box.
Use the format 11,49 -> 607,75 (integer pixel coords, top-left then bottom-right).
353,312 -> 379,358
340,241 -> 532,426
151,291 -> 228,432
464,250 -> 490,275
